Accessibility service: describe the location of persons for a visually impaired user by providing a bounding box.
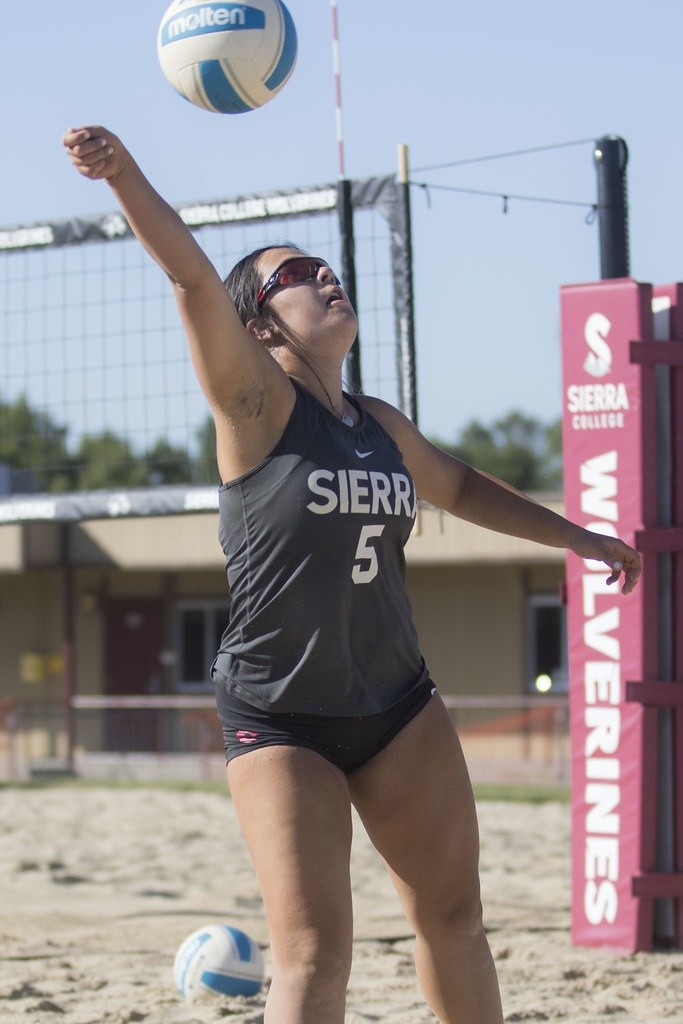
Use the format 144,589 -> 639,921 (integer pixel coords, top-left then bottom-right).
64,123 -> 640,1023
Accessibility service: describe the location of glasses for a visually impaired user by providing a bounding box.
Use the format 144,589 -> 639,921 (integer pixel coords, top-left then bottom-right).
258,257 -> 342,303
